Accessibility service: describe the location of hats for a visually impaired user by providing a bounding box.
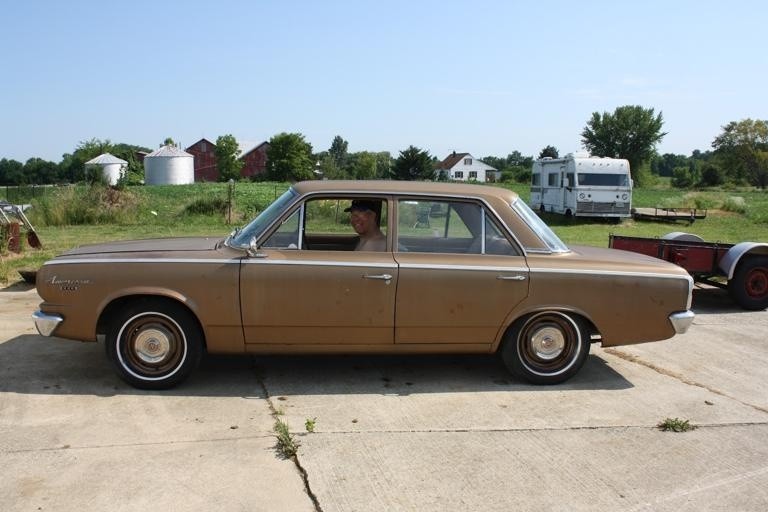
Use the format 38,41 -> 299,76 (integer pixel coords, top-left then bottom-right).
344,200 -> 380,212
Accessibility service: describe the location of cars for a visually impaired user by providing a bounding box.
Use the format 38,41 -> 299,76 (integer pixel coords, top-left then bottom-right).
31,181 -> 695,391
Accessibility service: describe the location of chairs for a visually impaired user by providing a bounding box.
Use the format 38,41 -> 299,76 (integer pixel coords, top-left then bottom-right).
469,234 -> 516,255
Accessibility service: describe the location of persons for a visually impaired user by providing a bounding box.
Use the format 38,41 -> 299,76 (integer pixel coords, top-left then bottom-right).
343,199 -> 387,252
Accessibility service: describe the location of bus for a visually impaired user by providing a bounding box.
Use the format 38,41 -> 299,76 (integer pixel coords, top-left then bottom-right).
529,155 -> 634,223
